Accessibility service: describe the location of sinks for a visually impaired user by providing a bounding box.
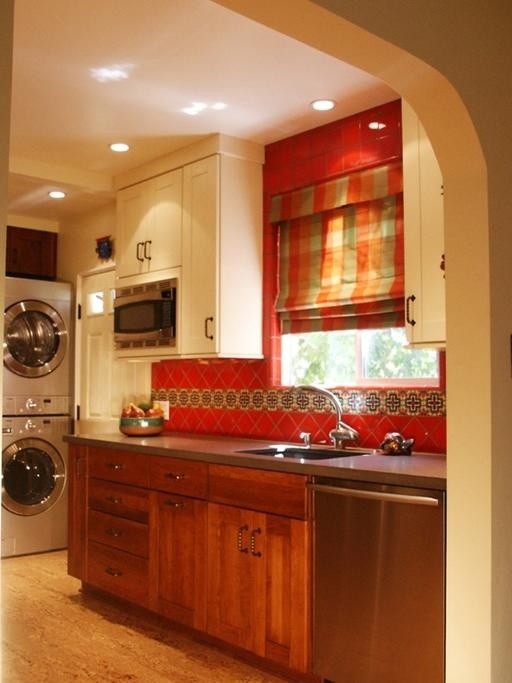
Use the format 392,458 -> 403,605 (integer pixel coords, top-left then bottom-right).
231,445 -> 377,461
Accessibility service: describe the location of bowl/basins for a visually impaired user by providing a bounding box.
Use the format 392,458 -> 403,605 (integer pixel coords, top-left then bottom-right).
119,418 -> 163,436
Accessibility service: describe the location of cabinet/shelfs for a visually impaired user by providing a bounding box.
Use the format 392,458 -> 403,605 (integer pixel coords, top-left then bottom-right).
206,464 -> 311,675
400,94 -> 447,353
176,155 -> 267,359
86,444 -> 156,614
148,456 -> 207,635
115,169 -> 183,277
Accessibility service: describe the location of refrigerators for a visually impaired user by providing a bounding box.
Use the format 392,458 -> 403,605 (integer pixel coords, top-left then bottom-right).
312,476 -> 446,682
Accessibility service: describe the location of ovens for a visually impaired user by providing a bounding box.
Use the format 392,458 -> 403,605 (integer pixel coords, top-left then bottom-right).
111,281 -> 177,350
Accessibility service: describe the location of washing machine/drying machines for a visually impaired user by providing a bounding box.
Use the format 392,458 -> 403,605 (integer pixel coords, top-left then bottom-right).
2,415 -> 74,559
1,275 -> 73,415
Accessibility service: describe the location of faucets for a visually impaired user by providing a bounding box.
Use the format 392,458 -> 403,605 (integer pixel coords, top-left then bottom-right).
288,384 -> 360,449
